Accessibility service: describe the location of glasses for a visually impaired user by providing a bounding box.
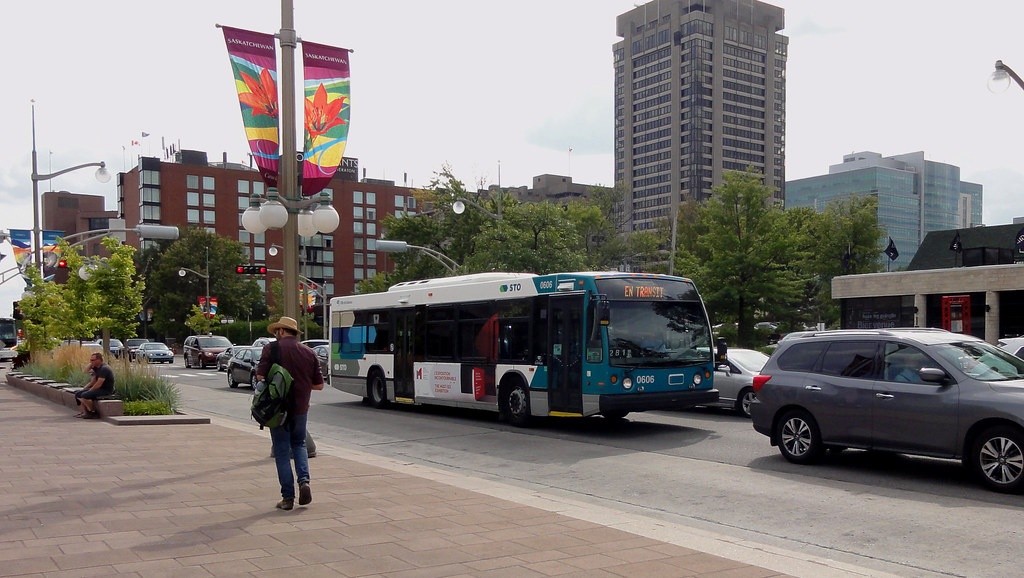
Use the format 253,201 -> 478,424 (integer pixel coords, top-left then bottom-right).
90,358 -> 99,361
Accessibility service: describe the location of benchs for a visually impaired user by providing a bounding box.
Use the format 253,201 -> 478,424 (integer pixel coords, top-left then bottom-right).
6,372 -> 123,417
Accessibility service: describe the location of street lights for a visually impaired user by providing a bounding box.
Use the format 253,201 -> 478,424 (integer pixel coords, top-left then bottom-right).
29,97 -> 112,287
178,246 -> 211,335
452,160 -> 504,232
242,0 -> 341,344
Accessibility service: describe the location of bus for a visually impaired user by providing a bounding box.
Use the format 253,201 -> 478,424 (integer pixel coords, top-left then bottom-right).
329,271 -> 720,426
0,317 -> 20,359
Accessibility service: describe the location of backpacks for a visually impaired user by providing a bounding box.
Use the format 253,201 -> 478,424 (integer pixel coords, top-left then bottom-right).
250,342 -> 296,432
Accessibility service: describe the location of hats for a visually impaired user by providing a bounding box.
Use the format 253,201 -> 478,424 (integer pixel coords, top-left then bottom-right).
268,317 -> 304,334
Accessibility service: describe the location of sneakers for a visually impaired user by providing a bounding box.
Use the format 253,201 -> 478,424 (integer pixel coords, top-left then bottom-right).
276,500 -> 293,510
299,483 -> 312,505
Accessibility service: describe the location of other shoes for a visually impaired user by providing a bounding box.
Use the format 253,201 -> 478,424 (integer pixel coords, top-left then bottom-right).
73,411 -> 100,419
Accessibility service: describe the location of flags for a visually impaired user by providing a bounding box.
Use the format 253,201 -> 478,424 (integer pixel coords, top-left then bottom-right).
842,243 -> 851,268
132,140 -> 139,145
142,132 -> 149,137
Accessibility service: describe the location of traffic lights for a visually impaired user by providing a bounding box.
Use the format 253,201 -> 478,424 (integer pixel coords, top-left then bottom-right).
237,265 -> 267,274
57,260 -> 69,268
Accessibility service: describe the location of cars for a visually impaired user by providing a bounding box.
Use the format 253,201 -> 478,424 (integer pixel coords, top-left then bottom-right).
124,338 -> 149,362
63,339 -> 125,360
183,335 -> 237,369
749,327 -> 1024,491
135,342 -> 175,364
695,347 -> 771,419
215,336 -> 330,391
972,335 -> 1024,377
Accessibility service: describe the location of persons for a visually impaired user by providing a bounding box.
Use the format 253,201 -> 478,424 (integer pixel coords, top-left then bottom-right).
1016,228 -> 1024,249
73,352 -> 115,419
256,316 -> 325,511
949,232 -> 962,254
894,352 -> 922,384
641,327 -> 665,351
883,236 -> 898,261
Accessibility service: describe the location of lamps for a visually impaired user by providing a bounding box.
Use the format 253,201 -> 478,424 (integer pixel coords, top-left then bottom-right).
985,305 -> 990,312
914,307 -> 918,313
